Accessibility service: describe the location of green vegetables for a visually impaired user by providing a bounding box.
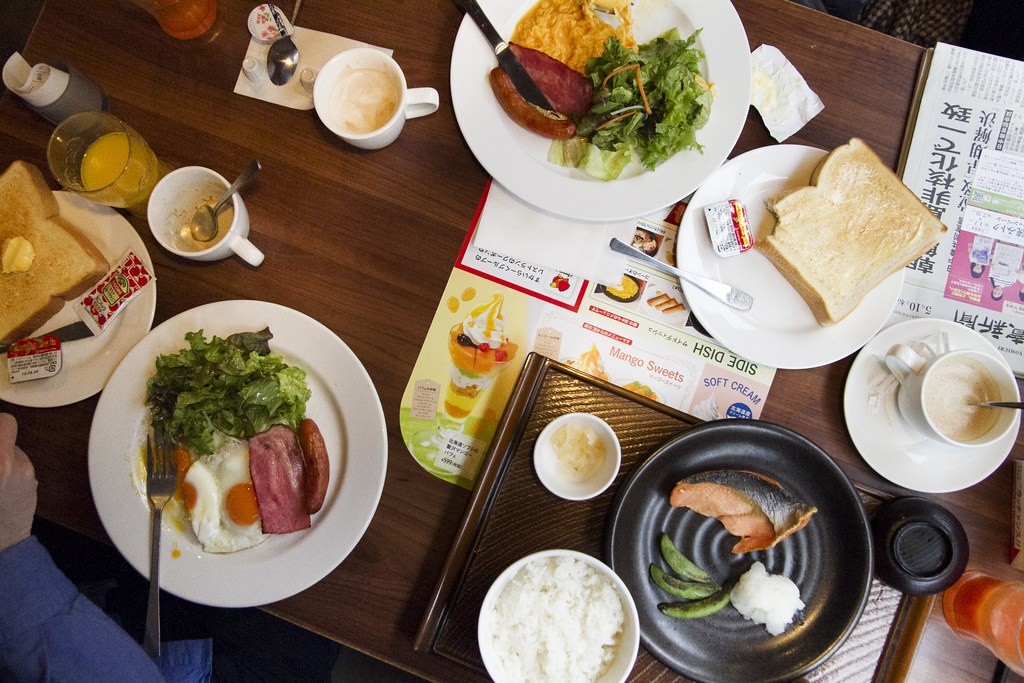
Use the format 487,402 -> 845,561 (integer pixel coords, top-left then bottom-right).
548,26 -> 712,179
142,326 -> 311,457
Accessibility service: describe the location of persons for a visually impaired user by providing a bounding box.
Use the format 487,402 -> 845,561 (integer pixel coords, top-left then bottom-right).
0,414 -> 342,683
988,241 -> 1024,302
967,235 -> 994,278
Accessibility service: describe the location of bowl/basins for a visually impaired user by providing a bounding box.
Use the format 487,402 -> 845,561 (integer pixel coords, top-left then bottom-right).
871,495 -> 970,597
534,413 -> 621,500
476,549 -> 640,683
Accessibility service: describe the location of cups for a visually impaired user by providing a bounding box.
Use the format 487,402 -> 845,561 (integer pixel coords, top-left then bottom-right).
148,167 -> 265,267
885,345 -> 1021,448
136,0 -> 215,39
313,47 -> 440,150
26,61 -> 102,125
47,110 -> 175,220
941,570 -> 1023,676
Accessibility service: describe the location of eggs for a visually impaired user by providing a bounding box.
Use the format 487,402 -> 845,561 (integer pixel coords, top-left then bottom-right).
182,441 -> 270,554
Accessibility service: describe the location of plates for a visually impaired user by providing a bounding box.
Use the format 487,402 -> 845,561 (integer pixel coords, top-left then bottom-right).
604,419 -> 872,682
676,145 -> 904,370
843,318 -> 1021,493
87,301 -> 389,608
0,191 -> 156,408
449,0 -> 751,221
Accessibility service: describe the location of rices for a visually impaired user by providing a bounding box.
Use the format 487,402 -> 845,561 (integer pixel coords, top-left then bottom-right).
487,556 -> 626,683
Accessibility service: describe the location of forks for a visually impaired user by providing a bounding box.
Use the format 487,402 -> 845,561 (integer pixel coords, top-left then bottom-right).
143,431 -> 177,657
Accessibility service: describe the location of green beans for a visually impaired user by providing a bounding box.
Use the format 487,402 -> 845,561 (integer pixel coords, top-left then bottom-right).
650,533 -> 732,619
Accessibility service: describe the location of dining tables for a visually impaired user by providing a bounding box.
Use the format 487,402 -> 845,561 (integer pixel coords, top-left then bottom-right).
1,0 -> 1023,683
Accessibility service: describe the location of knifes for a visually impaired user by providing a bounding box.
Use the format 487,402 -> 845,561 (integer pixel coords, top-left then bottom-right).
454,0 -> 560,117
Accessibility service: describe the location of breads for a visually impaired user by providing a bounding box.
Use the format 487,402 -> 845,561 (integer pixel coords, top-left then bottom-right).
754,137 -> 949,327
0,159 -> 111,353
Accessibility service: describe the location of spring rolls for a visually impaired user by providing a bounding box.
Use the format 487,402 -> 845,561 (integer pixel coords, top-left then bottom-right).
647,293 -> 686,315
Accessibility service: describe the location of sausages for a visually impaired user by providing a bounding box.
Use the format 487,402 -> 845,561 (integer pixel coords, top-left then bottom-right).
298,419 -> 329,515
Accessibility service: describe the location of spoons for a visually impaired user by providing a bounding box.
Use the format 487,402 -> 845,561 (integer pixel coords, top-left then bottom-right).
268,0 -> 302,86
190,159 -> 261,241
